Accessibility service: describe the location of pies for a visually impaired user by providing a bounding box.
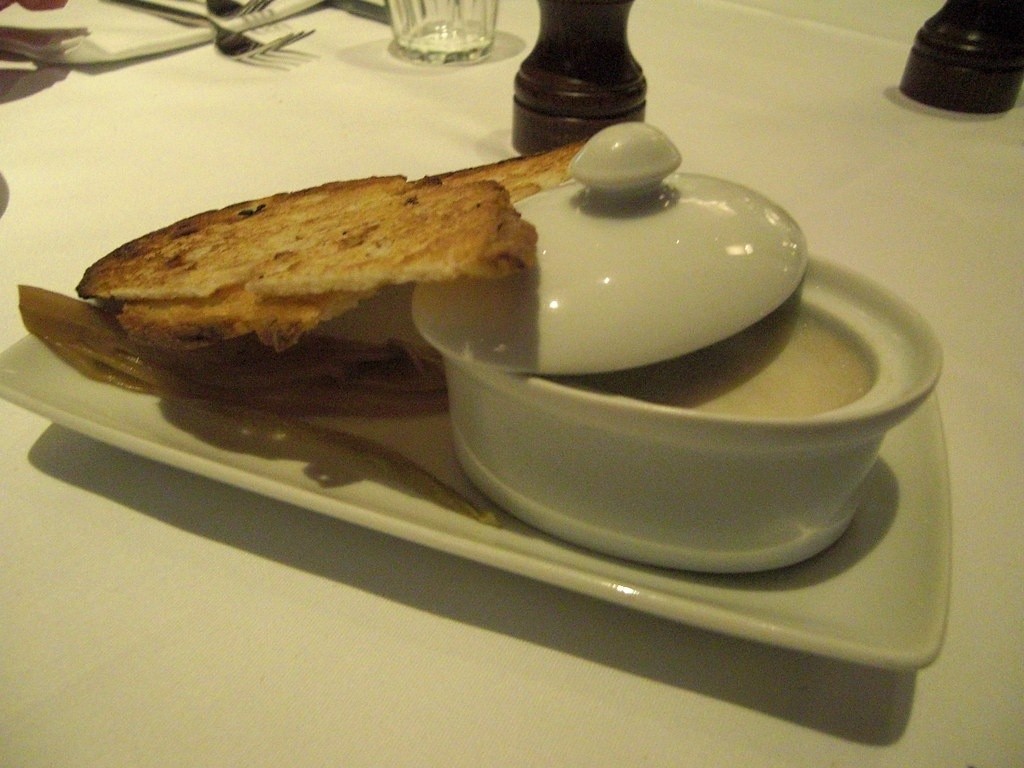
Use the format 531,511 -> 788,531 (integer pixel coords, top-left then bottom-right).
76,174 -> 535,299
118,139 -> 594,352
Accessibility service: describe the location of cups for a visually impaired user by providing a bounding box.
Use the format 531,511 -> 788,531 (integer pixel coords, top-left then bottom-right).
383,1 -> 501,65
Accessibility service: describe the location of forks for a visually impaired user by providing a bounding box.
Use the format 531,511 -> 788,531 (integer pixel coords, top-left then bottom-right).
100,0 -> 318,60
200,0 -> 275,21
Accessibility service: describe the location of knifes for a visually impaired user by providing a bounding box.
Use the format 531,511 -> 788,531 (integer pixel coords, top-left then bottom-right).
323,0 -> 391,25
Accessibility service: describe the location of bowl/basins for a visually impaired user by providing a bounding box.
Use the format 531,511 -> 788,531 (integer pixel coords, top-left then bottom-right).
409,122 -> 946,572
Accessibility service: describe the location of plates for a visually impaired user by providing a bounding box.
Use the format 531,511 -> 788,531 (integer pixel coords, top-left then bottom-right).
0,295 -> 954,674
